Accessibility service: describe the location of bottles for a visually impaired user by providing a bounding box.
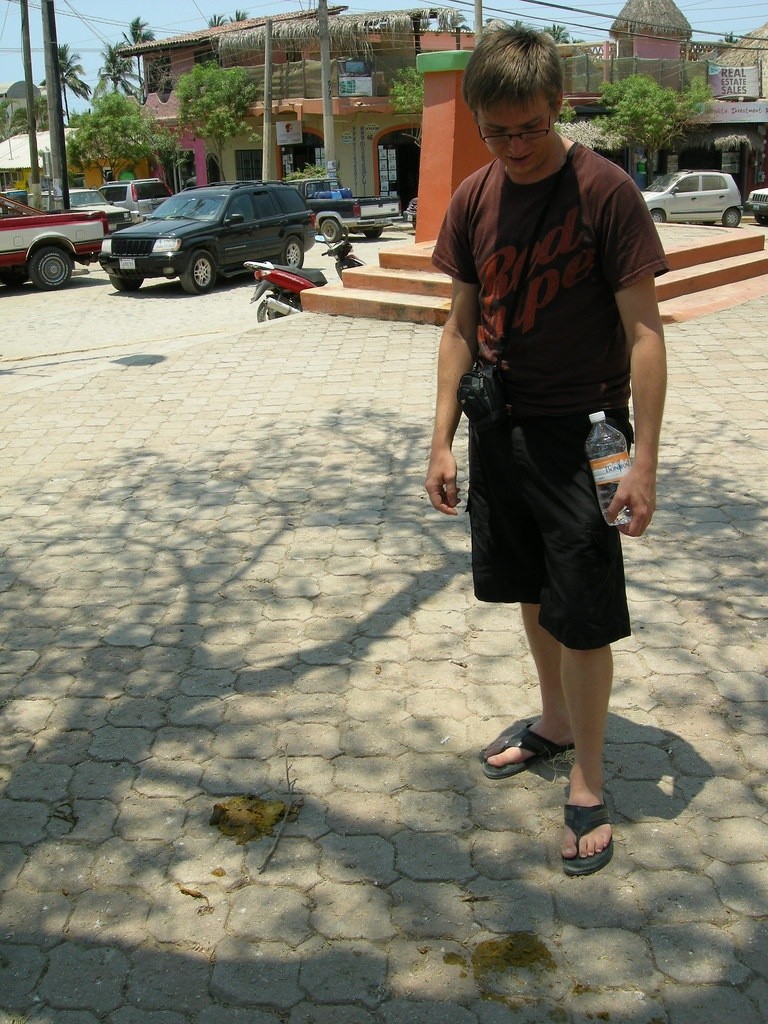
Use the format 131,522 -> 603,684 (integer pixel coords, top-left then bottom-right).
587,410 -> 636,526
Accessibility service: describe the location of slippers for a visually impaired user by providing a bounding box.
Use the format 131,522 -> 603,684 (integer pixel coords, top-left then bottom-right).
562,799 -> 615,876
478,721 -> 574,780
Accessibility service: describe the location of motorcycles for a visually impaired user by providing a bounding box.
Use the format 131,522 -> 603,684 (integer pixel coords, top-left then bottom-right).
244,225 -> 367,323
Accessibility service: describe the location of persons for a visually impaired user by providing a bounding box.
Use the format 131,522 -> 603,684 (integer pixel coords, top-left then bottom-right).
120,163 -> 137,179
424,28 -> 668,874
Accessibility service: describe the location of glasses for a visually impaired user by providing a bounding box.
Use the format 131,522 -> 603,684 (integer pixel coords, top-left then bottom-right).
476,104 -> 553,144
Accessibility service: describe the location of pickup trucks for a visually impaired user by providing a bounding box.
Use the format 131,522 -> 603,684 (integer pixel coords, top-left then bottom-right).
287,178 -> 401,243
0,196 -> 109,291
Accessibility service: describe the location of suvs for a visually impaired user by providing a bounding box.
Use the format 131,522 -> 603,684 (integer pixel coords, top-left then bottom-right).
93,178 -> 174,224
97,180 -> 317,296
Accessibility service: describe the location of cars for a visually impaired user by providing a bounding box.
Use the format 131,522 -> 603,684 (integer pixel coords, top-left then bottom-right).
743,188 -> 768,225
44,189 -> 134,234
640,168 -> 743,228
403,197 -> 418,229
